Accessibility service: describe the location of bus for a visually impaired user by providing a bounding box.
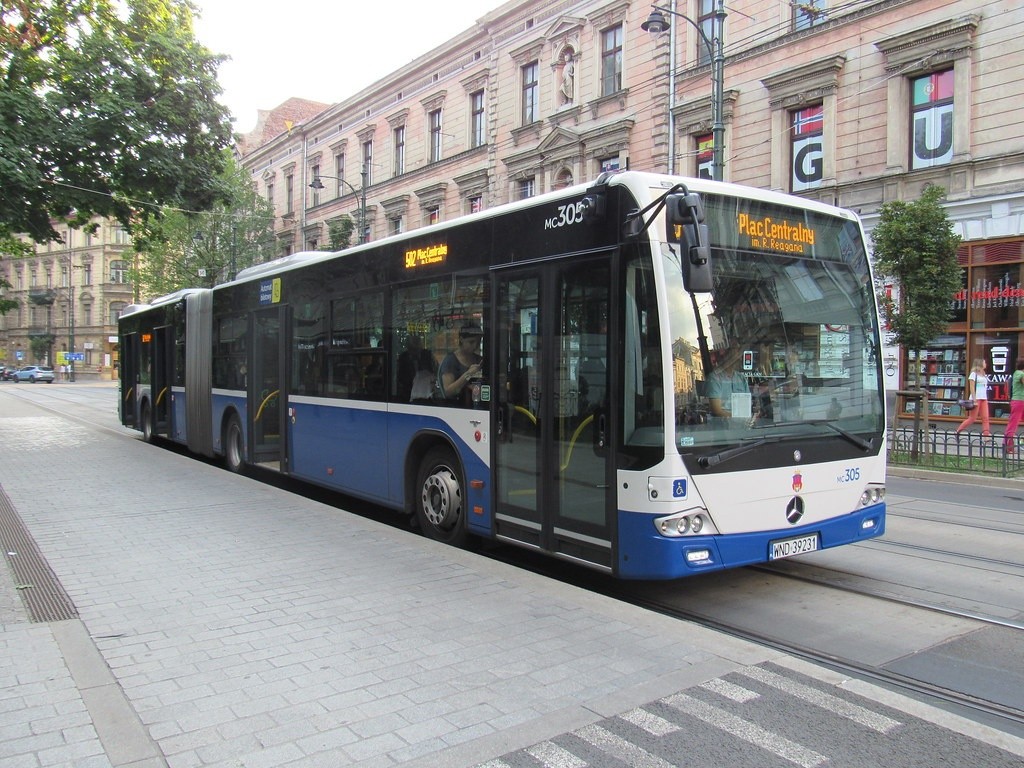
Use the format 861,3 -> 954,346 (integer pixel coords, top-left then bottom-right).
113,168 -> 889,581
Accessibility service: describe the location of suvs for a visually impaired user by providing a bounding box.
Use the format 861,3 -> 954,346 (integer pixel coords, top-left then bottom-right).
12,365 -> 55,384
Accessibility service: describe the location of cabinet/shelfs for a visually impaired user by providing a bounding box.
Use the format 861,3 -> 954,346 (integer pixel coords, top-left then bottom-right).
904,322 -> 968,417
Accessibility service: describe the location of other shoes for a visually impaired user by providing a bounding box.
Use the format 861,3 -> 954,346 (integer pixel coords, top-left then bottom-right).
984,439 -> 996,445
954,431 -> 960,442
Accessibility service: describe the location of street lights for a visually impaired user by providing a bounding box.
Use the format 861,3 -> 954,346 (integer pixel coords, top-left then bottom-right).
307,155 -> 370,243
193,221 -> 237,281
639,0 -> 728,182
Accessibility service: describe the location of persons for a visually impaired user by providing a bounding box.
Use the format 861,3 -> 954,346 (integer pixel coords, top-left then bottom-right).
707,336 -> 753,417
1002,360 -> 1024,453
48,358 -> 119,381
954,357 -> 996,445
0,359 -> 20,371
177,323 -> 186,344
397,321 -> 608,416
830,398 -> 841,419
559,52 -> 574,106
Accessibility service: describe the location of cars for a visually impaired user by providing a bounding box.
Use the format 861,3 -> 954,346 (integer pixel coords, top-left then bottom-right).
0,365 -> 21,382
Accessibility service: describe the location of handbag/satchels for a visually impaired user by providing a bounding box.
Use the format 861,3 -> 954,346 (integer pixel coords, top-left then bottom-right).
957,399 -> 975,411
